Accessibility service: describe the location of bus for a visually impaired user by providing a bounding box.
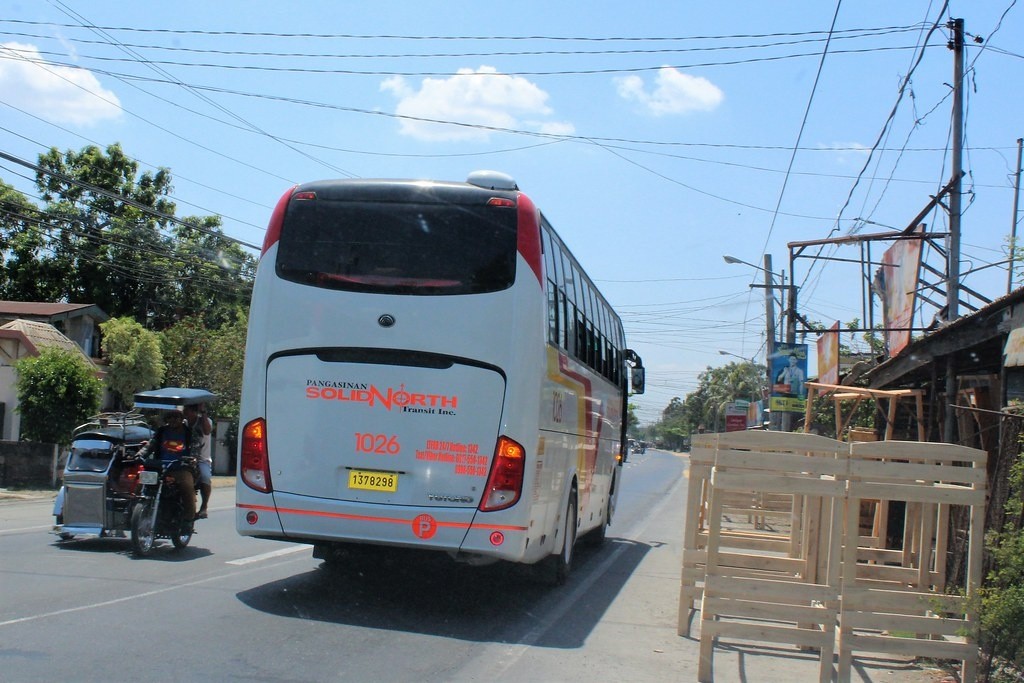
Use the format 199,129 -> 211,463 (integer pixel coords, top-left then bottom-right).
236,170 -> 649,588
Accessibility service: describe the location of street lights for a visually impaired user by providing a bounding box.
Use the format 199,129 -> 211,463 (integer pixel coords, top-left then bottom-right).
719,351 -> 756,402
722,255 -> 785,343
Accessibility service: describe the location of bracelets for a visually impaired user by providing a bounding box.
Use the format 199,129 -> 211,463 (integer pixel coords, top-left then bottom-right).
200,411 -> 206,413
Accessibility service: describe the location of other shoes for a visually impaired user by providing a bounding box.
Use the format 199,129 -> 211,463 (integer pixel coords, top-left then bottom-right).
194,511 -> 208,519
182,506 -> 196,521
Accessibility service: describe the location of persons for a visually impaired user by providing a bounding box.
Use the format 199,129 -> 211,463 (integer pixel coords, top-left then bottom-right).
777,352 -> 804,398
127,411 -> 196,536
183,402 -> 214,518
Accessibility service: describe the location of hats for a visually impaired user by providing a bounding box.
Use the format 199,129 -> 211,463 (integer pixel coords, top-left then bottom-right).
163,409 -> 186,419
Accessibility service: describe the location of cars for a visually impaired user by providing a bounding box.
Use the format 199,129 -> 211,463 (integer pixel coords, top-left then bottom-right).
630,445 -> 644,455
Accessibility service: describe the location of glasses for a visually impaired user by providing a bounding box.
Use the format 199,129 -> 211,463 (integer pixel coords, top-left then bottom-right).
166,412 -> 182,420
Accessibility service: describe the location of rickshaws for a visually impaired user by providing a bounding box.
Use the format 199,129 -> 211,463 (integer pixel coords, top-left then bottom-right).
48,389 -> 221,559
